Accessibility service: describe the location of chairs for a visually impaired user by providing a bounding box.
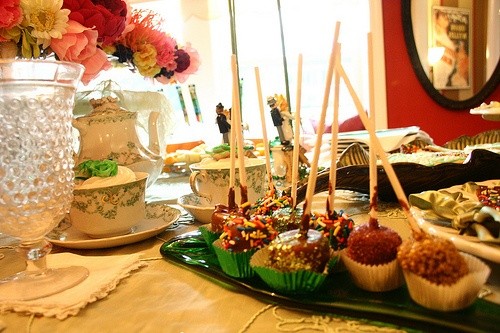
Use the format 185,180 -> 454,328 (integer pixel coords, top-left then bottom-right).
244,139 -> 264,151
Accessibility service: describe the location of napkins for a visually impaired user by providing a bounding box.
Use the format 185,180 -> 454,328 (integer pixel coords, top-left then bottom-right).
0,252 -> 148,321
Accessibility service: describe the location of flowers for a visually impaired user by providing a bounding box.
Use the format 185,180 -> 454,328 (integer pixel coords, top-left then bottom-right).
0,0 -> 201,86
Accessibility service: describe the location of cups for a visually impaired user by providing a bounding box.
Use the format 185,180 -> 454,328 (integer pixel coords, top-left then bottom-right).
188,159 -> 273,208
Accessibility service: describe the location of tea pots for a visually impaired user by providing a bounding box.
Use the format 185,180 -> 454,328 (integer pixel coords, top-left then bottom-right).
72,96 -> 164,188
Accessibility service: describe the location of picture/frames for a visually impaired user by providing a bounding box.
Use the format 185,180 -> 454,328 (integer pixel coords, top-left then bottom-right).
432,5 -> 473,90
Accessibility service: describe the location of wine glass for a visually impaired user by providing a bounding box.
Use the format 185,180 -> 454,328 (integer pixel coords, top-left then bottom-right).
0,60 -> 90,301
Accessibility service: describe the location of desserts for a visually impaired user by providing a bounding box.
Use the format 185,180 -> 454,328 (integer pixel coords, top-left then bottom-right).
200,144 -> 261,168
74,159 -> 135,189
202,186 -> 491,311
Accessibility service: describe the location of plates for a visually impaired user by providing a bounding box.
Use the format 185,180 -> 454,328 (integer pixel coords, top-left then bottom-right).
178,191 -> 283,222
410,179 -> 500,264
43,203 -> 181,249
160,230 -> 500,333
67,172 -> 149,238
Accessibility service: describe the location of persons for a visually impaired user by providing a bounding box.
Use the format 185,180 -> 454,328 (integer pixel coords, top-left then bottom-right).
215,102 -> 245,147
267,96 -> 296,145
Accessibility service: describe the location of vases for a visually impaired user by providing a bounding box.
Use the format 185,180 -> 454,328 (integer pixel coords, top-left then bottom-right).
0,57 -> 90,302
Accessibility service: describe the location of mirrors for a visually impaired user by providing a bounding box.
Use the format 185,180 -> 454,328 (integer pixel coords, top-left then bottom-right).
401,0 -> 500,113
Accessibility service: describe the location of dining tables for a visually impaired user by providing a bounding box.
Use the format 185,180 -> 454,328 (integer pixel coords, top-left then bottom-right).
0,174 -> 500,333
